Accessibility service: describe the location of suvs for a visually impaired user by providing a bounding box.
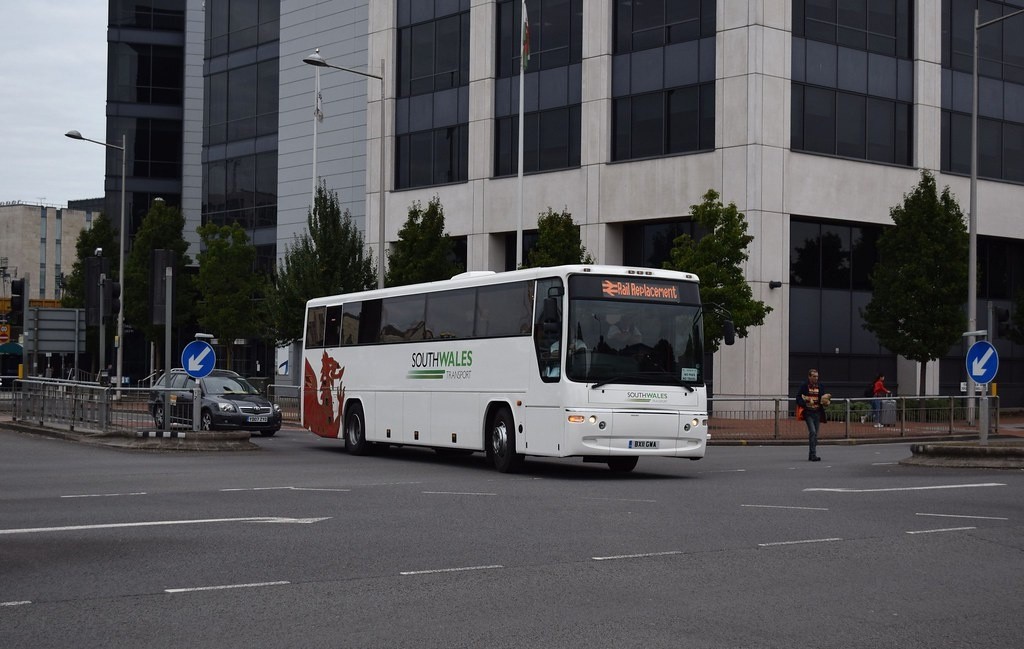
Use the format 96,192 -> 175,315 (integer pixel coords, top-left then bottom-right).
145,367 -> 283,437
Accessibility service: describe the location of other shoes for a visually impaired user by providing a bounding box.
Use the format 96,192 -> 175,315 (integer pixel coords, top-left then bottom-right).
874,422 -> 884,427
861,415 -> 866,423
814,455 -> 821,460
809,456 -> 816,461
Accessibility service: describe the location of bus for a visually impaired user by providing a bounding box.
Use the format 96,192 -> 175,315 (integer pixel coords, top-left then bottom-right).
298,260 -> 736,481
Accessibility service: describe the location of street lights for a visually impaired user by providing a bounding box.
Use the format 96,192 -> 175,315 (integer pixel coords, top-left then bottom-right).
301,52 -> 388,290
64,129 -> 127,405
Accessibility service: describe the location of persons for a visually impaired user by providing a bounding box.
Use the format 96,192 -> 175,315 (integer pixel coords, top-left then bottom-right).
608,314 -> 647,345
796,368 -> 832,462
550,323 -> 588,377
861,373 -> 891,428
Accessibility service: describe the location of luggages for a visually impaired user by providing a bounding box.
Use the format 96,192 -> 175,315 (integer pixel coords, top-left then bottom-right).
881,392 -> 896,426
